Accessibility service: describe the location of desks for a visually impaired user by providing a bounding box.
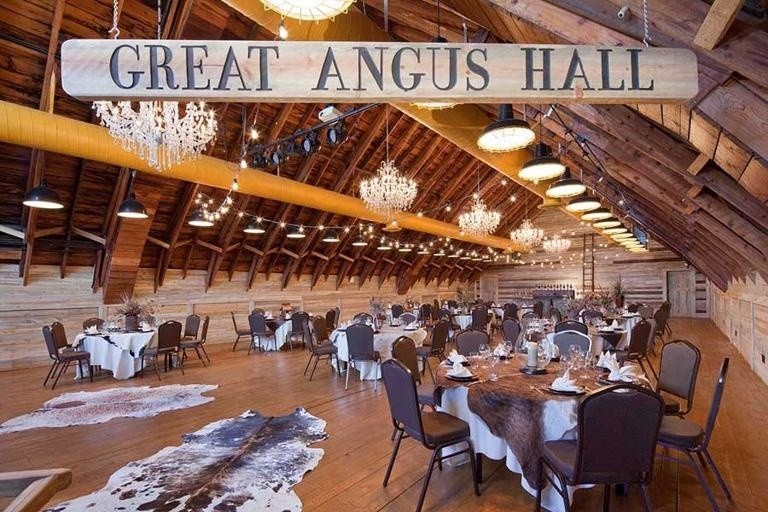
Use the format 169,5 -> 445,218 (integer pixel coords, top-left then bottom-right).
253,314 -> 313,351
436,351 -> 658,502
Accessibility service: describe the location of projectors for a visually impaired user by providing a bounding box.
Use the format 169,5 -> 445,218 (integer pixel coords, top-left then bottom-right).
318,106 -> 344,125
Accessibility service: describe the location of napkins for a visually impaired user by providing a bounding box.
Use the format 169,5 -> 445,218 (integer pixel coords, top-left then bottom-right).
447,361 -> 474,378
448,349 -> 468,363
552,365 -> 586,394
597,351 -> 619,372
609,365 -> 649,382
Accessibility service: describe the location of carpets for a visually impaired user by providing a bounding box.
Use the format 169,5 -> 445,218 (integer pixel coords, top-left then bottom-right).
0,384 -> 219,434
46,406 -> 329,511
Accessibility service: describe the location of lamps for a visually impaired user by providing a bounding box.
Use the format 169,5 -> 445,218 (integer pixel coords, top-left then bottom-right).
251,117 -> 346,170
261,0 -> 359,24
187,194 -> 214,227
509,190 -> 544,249
458,161 -> 501,239
474,103 -> 535,156
286,216 -> 306,238
22,150 -> 64,209
544,209 -> 571,254
360,106 -> 418,220
242,219 -> 266,234
321,221 -> 492,272
520,141 -> 647,254
116,169 -> 149,219
91,1 -> 220,174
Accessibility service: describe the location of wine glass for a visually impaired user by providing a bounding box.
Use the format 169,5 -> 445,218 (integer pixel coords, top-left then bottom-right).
469,341 -> 602,381
585,315 -> 602,328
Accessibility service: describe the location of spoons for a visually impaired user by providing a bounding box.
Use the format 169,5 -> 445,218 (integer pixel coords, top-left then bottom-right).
528,385 -> 544,395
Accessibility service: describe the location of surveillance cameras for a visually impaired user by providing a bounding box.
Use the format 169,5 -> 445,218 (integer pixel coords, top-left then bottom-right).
618,6 -> 629,19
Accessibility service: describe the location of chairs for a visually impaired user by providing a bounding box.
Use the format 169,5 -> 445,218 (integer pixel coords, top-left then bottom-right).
414,319 -> 448,387
651,358 -> 729,509
230,311 -> 256,352
655,338 -> 708,470
380,357 -> 482,510
41,314 -> 211,393
325,295 -> 673,357
312,315 -> 329,344
247,314 -> 278,357
286,311 -> 312,353
252,308 -> 266,316
609,321 -> 659,385
344,323 -> 383,393
537,383 -> 666,512
302,320 -> 341,381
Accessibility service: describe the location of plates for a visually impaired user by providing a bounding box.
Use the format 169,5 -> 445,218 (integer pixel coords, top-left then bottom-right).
541,382 -> 587,395
452,313 -> 460,315
339,314 -> 419,333
622,315 -> 632,318
284,318 -> 290,321
599,374 -> 632,385
445,359 -> 469,366
600,330 -> 614,333
83,324 -> 154,338
615,328 -> 623,330
443,371 -> 479,381
265,318 -> 273,321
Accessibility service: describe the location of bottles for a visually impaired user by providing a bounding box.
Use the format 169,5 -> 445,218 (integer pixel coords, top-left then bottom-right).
535,283 -> 572,290
